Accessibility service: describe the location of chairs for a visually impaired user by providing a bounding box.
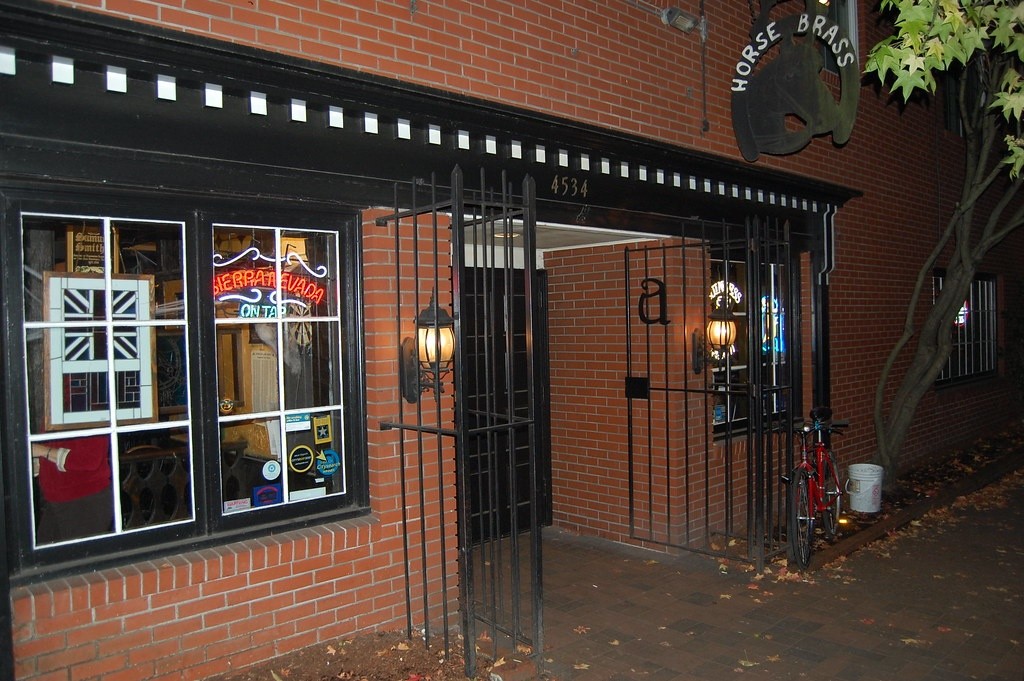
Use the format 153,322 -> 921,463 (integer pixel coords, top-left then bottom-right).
102,439 -> 248,530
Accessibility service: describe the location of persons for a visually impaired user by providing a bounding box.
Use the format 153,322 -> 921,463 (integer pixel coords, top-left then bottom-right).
31,376 -> 114,548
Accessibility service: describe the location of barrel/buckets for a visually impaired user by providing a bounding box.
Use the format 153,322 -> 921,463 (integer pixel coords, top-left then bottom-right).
845,463 -> 883,512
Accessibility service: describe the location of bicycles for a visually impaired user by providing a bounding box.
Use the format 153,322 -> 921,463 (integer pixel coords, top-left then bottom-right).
764,407 -> 844,574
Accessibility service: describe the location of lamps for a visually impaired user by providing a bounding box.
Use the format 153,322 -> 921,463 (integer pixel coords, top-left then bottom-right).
400,286 -> 455,404
692,291 -> 737,375
667,7 -> 699,34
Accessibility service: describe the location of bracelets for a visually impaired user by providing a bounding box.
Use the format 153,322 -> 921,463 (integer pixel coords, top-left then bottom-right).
46,447 -> 53,460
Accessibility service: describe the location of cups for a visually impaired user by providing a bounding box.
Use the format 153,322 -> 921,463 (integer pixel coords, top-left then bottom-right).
715,406 -> 725,421
